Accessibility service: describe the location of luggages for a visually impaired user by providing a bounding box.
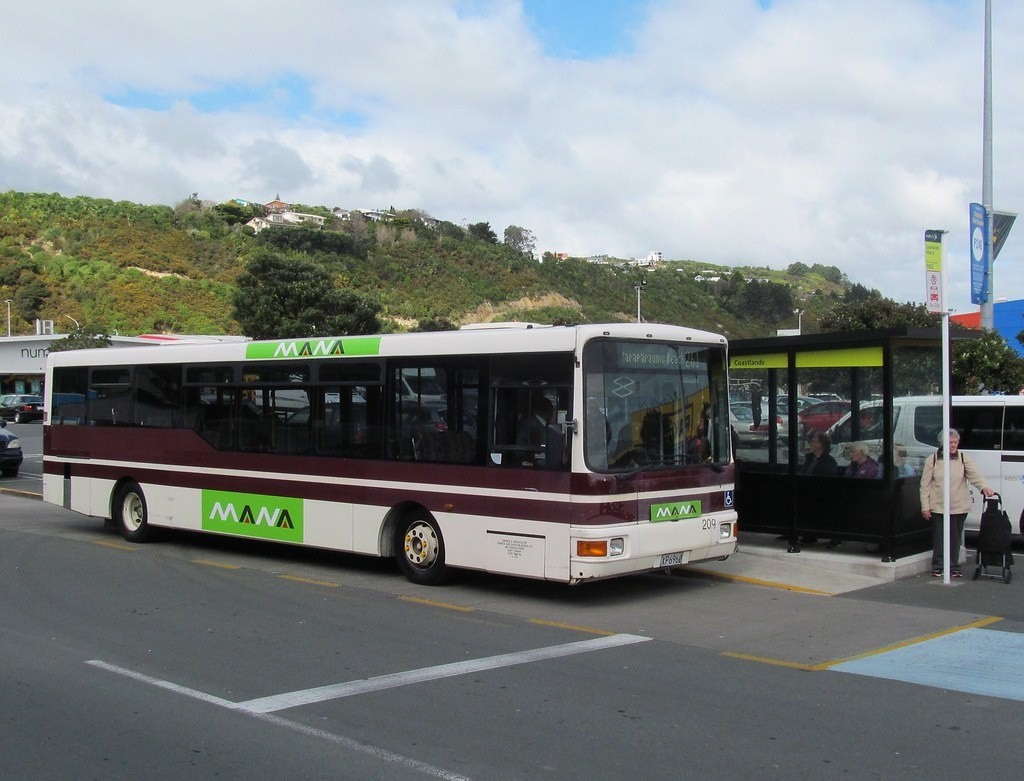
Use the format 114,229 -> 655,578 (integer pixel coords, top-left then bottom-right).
974,492 -> 1015,584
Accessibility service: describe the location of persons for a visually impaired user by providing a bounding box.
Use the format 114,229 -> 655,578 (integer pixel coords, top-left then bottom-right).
516,383 -> 561,468
799,432 -> 838,477
919,429 -> 993,576
412,409 -> 450,462
877,443 -> 916,478
751,381 -> 762,431
842,442 -> 879,478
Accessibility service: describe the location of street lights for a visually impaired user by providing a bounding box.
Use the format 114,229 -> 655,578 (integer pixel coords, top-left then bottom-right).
794,308 -> 806,335
632,279 -> 646,323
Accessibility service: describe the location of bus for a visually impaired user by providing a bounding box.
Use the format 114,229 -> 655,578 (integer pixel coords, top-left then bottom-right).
41,322 -> 738,587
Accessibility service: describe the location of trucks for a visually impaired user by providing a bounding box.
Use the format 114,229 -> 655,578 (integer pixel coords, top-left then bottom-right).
254,373 -> 368,426
397,368 -> 446,410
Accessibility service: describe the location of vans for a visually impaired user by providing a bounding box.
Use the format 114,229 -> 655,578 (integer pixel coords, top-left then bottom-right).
823,395 -> 1024,534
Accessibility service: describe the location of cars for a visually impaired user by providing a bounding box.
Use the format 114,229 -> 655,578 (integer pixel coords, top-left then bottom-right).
730,393 -> 842,445
0,426 -> 23,478
799,399 -> 877,432
730,407 -> 782,447
0,394 -> 44,424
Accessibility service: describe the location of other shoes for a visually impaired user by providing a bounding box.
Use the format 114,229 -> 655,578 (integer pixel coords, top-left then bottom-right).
775,535 -> 789,542
951,568 -> 963,577
800,537 -> 818,543
930,568 -> 942,577
824,541 -> 842,549
